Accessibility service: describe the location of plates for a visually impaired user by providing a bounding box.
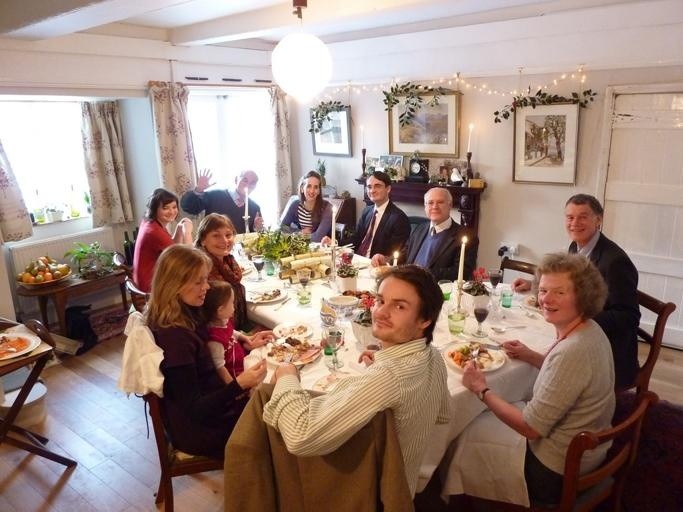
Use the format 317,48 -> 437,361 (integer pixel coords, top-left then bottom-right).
445,341 -> 506,372
17,270 -> 73,290
262,323 -> 321,366
0,334 -> 41,361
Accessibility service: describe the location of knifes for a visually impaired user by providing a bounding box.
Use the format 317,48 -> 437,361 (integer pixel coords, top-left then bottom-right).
471,341 -> 510,352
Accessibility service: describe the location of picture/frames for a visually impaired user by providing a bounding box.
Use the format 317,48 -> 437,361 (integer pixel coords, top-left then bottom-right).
378,155 -> 403,177
511,102 -> 580,184
387,91 -> 460,158
309,108 -> 353,158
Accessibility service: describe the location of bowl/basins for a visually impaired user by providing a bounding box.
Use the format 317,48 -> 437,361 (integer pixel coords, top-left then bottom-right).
328,296 -> 359,317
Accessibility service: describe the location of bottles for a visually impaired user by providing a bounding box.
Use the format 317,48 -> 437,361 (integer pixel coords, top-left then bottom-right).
123,231 -> 134,265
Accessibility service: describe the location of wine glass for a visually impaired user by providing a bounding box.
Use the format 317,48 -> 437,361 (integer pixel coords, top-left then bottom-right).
488,269 -> 503,289
472,303 -> 489,337
448,313 -> 465,337
298,271 -> 310,287
325,327 -> 344,371
252,255 -> 265,280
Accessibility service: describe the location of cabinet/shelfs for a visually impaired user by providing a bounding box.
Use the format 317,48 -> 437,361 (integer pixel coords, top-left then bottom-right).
354,176 -> 487,281
279,194 -> 357,245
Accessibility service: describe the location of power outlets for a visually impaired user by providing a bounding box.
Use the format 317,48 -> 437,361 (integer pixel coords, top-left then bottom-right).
499,241 -> 520,258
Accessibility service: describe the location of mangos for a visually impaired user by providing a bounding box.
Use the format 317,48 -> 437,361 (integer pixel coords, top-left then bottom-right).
57,263 -> 68,275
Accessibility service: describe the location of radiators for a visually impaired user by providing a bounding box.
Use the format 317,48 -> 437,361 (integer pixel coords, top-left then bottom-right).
8,226 -> 116,314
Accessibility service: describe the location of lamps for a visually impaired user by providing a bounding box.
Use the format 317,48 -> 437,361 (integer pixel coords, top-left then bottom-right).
271,0 -> 334,107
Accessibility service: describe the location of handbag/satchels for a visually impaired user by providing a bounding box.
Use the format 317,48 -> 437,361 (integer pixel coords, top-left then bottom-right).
65,304 -> 98,355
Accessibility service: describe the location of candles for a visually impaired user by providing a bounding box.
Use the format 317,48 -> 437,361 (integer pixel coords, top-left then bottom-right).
457,235 -> 469,283
467,124 -> 472,151
361,126 -> 365,149
331,204 -> 337,246
243,186 -> 249,217
393,250 -> 399,268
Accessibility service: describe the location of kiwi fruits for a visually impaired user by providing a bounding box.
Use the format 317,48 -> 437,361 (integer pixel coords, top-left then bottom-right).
16,273 -> 23,281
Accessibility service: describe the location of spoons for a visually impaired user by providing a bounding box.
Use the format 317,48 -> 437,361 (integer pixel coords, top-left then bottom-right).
491,326 -> 528,333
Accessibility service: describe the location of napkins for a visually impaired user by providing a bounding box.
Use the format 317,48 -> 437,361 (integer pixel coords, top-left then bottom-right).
438,400 -> 531,509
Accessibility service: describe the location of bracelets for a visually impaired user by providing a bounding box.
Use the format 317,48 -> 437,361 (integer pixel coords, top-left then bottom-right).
274,361 -> 298,381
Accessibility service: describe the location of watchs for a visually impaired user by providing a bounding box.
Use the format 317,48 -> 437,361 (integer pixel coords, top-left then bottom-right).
478,387 -> 490,401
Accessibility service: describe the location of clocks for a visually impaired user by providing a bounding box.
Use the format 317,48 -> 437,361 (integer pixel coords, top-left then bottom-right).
404,159 -> 429,183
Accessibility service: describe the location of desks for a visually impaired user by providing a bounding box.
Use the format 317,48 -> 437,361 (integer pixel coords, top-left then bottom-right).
0,322 -> 77,468
15,266 -> 127,331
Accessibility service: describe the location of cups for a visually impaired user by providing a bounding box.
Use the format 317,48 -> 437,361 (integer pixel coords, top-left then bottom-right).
297,287 -> 313,305
502,294 -> 512,308
438,280 -> 452,301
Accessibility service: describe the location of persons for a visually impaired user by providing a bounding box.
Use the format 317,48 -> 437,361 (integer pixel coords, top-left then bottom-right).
142,213 -> 280,461
461,249 -> 617,506
180,167 -> 265,234
279,170 -> 334,243
370,187 -> 476,283
132,188 -> 193,294
261,264 -> 455,501
511,193 -> 641,390
320,171 -> 411,260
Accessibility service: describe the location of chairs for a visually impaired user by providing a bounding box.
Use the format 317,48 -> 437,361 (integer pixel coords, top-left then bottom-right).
137,389 -> 224,512
534,389 -> 660,510
611,291 -> 676,417
257,383 -> 393,510
127,278 -> 151,314
495,257 -> 541,288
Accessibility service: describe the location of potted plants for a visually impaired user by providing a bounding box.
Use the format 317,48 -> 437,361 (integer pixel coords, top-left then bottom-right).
315,158 -> 326,186
62,240 -> 114,275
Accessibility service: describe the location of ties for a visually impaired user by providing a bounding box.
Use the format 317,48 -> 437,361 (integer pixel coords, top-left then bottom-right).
238,199 -> 244,207
355,210 -> 378,256
